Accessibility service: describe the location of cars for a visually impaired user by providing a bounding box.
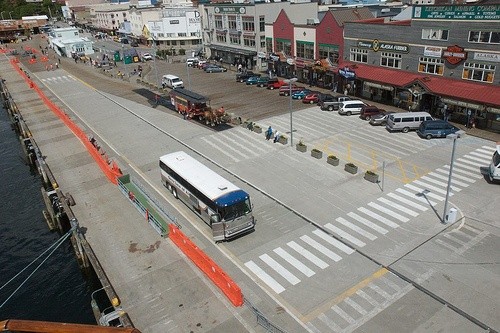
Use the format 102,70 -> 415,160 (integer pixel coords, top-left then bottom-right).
370,110 -> 393,126
302,91 -> 333,104
360,106 -> 386,121
279,83 -> 305,97
246,76 -> 289,90
291,89 -> 312,99
186,58 -> 227,73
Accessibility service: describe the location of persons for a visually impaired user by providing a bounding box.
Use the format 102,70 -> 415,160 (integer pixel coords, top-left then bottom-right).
265,126 -> 272,140
40,45 -> 49,57
46,58 -> 61,71
334,87 -> 337,94
344,89 -> 348,96
273,130 -> 279,143
239,64 -> 242,72
182,108 -> 187,120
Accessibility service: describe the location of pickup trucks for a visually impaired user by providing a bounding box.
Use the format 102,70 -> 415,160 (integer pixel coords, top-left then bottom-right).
320,96 -> 353,111
236,71 -> 262,83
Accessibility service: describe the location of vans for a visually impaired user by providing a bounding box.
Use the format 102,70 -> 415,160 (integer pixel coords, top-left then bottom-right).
385,112 -> 435,133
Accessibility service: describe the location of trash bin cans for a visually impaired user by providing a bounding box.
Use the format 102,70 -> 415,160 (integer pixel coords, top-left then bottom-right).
114,53 -> 120,62
122,54 -> 132,65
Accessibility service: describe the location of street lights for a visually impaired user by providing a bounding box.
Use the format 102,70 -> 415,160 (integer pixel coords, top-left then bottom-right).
150,35 -> 159,85
120,44 -> 130,77
442,129 -> 466,224
284,77 -> 299,147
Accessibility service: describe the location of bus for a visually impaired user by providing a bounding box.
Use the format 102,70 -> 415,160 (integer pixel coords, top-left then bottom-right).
158,150 -> 255,244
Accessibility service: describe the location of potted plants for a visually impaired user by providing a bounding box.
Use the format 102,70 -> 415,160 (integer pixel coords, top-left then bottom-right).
279,135 -> 288,144
365,171 -> 378,183
296,140 -> 307,152
345,163 -> 358,174
311,149 -> 322,159
327,155 -> 339,166
254,126 -> 262,133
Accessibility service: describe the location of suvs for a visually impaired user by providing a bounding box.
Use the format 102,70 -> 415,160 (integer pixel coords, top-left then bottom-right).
489,144 -> 500,183
338,99 -> 369,116
162,74 -> 184,90
418,120 -> 460,139
